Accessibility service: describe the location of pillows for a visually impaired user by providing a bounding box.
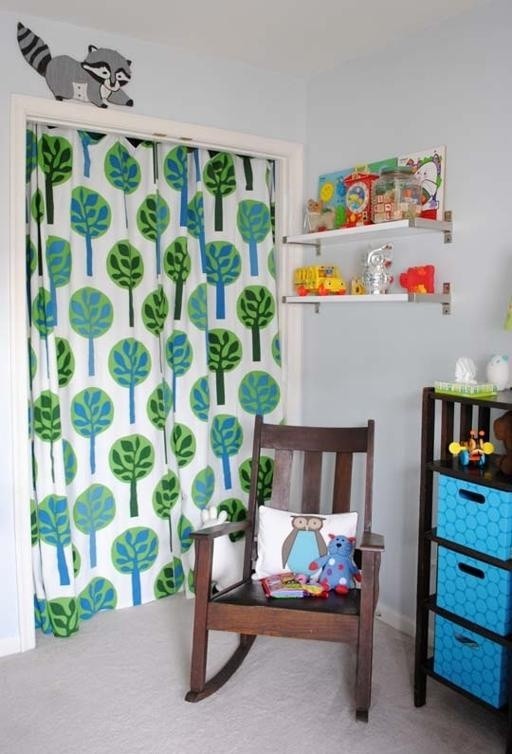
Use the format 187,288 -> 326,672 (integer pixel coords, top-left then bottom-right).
250,504 -> 358,582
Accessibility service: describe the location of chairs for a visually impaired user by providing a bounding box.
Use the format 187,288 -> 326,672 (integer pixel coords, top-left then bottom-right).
184,415 -> 386,725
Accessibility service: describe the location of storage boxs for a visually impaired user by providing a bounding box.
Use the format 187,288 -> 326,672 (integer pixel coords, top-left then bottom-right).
436,471 -> 512,561
433,612 -> 511,710
434,545 -> 512,637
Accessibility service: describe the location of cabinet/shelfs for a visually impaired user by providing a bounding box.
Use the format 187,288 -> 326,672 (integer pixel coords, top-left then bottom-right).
413,386 -> 512,753
280,210 -> 454,315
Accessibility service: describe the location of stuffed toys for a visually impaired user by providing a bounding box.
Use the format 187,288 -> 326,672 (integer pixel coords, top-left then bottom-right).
455,357 -> 476,384
493,410 -> 512,474
308,533 -> 362,596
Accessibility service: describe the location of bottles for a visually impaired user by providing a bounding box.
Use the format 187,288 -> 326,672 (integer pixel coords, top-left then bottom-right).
371,166 -> 422,225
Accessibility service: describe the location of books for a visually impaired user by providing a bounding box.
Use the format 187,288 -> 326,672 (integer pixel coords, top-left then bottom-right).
261,574 -> 305,600
